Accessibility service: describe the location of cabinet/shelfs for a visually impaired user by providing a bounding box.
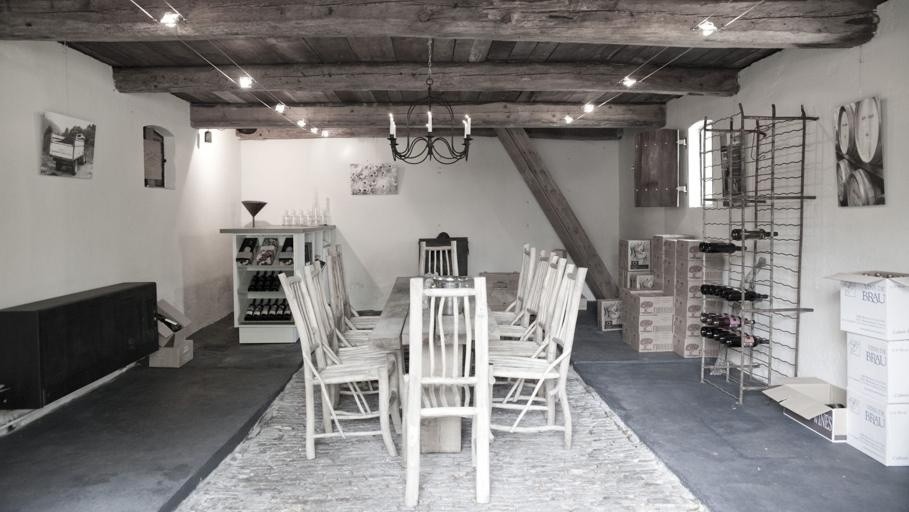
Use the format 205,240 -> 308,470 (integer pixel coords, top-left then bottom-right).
0,282 -> 159,409
699,103 -> 819,405
634,129 -> 687,207
220,225 -> 336,344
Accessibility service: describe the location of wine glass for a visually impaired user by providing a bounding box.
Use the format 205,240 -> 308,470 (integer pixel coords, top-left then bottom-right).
240,199 -> 268,228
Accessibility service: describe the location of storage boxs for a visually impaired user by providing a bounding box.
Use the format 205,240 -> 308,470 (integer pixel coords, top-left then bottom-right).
823,270 -> 909,342
597,234 -> 730,358
846,387 -> 909,467
149,299 -> 194,368
762,377 -> 846,443
846,332 -> 908,404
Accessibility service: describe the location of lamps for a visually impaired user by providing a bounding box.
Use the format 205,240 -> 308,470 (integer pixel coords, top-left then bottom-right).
388,39 -> 474,164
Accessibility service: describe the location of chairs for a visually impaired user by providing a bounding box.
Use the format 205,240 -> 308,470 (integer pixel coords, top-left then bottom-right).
277,270 -> 402,459
404,277 -> 490,507
489,264 -> 589,451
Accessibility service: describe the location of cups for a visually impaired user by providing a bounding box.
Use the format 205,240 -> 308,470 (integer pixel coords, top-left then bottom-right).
279,208 -> 330,227
428,274 -> 473,316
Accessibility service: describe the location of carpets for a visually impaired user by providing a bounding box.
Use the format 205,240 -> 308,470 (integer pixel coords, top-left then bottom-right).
172,347 -> 712,512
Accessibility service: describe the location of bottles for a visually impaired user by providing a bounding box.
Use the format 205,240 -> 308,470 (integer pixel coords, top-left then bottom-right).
155,313 -> 181,332
243,298 -> 291,321
247,271 -> 283,292
698,282 -> 772,350
730,227 -> 779,241
311,192 -> 321,226
698,241 -> 749,254
